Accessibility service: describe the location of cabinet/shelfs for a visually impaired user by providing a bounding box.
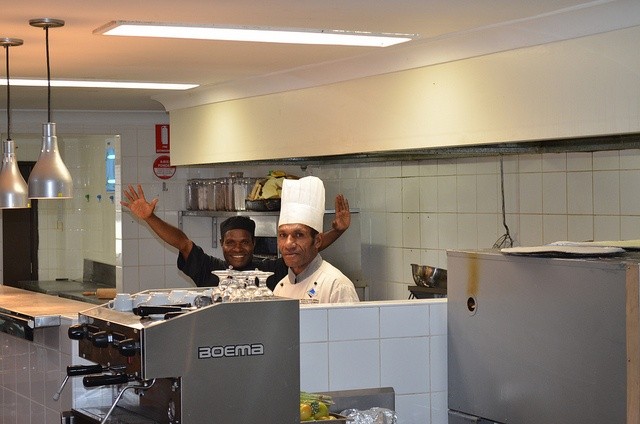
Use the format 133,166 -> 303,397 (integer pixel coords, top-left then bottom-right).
173,209 -> 363,217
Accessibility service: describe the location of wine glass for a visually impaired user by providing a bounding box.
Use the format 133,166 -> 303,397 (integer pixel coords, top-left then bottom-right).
254,272 -> 274,297
222,271 -> 238,303
212,269 -> 232,303
229,272 -> 251,302
244,271 -> 262,299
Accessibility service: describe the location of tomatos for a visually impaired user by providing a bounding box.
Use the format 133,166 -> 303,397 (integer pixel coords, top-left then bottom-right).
300,403 -> 311,421
312,401 -> 330,419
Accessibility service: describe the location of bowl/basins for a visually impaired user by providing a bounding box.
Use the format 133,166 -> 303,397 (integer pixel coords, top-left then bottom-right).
410,263 -> 447,289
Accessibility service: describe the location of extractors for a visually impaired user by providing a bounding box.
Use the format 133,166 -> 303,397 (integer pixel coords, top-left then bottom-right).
252,216 -> 281,259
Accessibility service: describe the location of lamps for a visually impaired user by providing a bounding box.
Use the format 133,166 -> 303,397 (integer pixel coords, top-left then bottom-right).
92,20 -> 422,49
0,78 -> 200,90
29,19 -> 73,198
0,38 -> 32,208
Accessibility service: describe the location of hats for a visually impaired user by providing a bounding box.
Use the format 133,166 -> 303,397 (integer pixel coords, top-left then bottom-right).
220,215 -> 255,237
278,175 -> 326,234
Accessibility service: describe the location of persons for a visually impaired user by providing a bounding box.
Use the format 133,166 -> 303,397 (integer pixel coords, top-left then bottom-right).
272,208 -> 360,305
120,182 -> 351,293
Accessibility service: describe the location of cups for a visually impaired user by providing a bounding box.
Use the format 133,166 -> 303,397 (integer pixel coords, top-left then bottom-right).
134,293 -> 150,308
147,292 -> 169,306
111,293 -> 133,312
169,288 -> 212,306
